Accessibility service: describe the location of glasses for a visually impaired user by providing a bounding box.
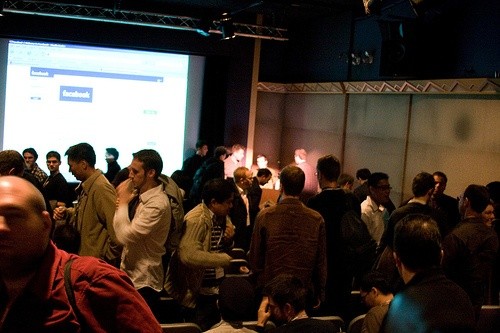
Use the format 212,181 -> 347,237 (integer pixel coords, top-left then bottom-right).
314,169 -> 318,176
456,196 -> 466,201
375,185 -> 392,191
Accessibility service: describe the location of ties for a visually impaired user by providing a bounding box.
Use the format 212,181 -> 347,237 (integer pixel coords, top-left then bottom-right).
130,197 -> 139,220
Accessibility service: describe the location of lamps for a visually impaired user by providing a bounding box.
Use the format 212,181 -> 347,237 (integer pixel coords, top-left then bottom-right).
221,19 -> 234,39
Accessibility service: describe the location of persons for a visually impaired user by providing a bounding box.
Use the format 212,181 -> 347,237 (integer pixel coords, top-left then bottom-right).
197,276 -> 262,333
0,174 -> 165,333
356,268 -> 402,333
380,215 -> 494,333
256,275 -> 347,333
0,141 -> 500,333
